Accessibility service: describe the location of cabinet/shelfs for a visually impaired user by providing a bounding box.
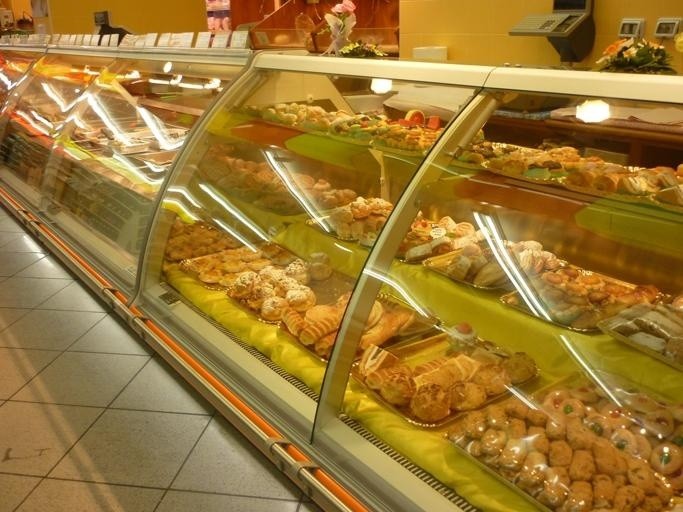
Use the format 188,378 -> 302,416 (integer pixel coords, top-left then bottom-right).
0,43 -> 683,512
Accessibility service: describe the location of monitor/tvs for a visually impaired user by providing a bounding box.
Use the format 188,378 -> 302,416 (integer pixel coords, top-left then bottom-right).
551,0 -> 592,12
95,10 -> 111,28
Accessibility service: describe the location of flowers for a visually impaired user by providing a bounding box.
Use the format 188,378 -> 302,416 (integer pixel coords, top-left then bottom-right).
322,0 -> 358,56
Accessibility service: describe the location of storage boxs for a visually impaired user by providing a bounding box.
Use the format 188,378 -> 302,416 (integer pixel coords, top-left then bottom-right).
313,0 -> 399,58
250,0 -> 370,58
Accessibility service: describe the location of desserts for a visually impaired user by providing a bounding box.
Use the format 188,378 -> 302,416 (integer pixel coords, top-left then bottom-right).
203,142 -> 682,366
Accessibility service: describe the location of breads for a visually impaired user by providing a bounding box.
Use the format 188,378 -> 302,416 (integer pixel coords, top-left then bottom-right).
165,102 -> 683,207
180,243 -> 683,511
168,216 -> 240,262
